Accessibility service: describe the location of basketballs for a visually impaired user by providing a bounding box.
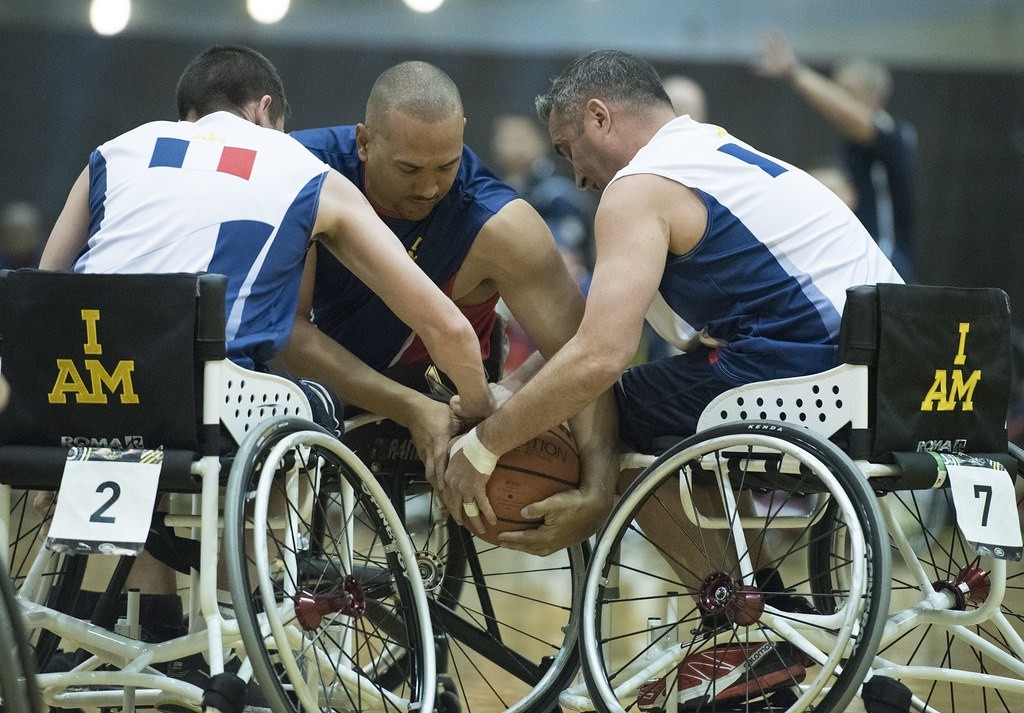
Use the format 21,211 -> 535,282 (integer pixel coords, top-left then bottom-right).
453,420 -> 581,545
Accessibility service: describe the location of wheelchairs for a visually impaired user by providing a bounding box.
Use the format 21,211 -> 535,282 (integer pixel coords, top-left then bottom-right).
575,282 -> 1023,713
0,259 -> 593,713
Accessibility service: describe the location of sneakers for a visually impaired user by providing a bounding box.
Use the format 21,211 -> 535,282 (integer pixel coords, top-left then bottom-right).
146,648 -> 305,713
637,644 -> 811,713
766,594 -> 825,667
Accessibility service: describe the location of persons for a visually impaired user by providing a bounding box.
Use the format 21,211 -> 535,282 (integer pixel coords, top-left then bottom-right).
35,42 -> 919,713
751,31 -> 916,283
